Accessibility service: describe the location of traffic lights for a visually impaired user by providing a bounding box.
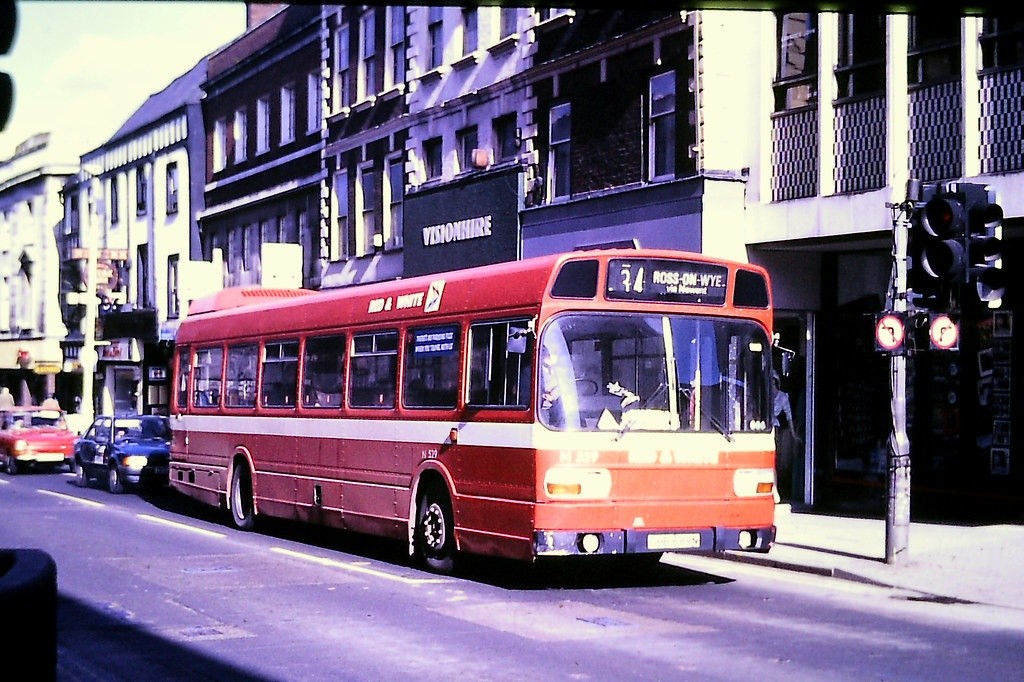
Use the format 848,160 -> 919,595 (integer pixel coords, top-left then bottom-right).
965,184 -> 1004,305
919,180 -> 970,289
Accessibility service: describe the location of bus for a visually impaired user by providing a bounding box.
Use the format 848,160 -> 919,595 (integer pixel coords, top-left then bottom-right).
168,248 -> 798,576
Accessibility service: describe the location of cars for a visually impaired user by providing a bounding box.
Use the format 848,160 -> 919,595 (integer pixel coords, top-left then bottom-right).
73,416 -> 169,495
0,406 -> 80,476
